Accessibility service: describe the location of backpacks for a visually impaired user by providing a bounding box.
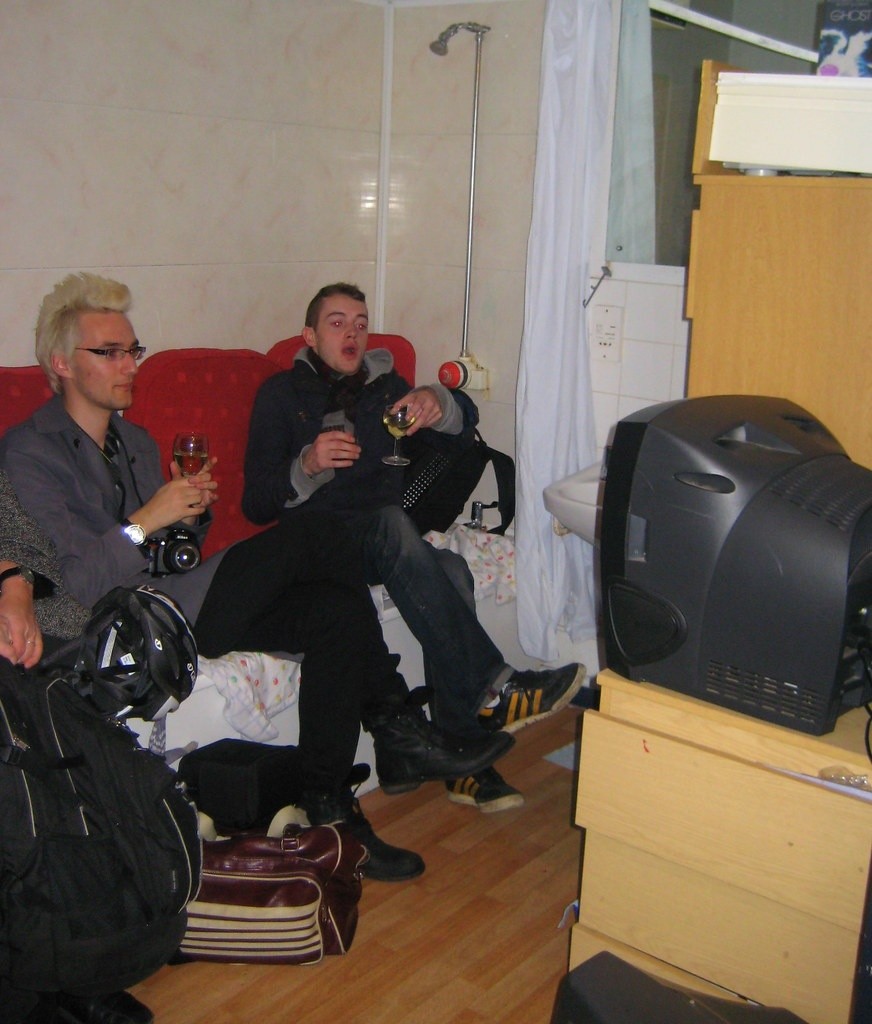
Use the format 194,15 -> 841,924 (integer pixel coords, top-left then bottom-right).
0,656 -> 204,995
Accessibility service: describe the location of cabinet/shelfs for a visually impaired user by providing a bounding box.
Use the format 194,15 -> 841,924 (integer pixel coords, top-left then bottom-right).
567,666 -> 872,1024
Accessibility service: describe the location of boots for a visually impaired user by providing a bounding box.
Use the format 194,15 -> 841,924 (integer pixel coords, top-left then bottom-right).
257,755 -> 425,881
372,686 -> 515,795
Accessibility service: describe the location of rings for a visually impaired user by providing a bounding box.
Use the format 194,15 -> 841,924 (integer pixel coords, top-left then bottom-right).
26,640 -> 35,644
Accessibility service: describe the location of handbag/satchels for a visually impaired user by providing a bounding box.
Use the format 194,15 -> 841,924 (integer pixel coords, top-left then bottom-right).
177,821 -> 370,965
400,427 -> 516,536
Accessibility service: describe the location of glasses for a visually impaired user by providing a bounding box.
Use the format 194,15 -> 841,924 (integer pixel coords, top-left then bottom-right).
75,346 -> 146,361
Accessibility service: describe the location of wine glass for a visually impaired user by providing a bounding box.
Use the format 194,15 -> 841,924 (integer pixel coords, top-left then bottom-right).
381,404 -> 416,466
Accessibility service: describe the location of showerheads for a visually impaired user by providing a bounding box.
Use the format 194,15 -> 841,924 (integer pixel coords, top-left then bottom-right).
430,38 -> 448,55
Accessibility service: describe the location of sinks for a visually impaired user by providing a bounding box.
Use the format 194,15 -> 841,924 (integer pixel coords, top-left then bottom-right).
543,460 -> 608,550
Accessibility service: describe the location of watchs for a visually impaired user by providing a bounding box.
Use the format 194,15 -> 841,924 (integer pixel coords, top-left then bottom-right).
0,565 -> 35,584
120,518 -> 150,558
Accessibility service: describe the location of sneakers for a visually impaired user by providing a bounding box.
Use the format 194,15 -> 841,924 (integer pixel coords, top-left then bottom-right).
445,764 -> 524,812
475,662 -> 586,733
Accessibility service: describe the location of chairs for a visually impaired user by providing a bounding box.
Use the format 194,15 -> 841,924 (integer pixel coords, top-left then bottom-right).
0,333 -> 416,560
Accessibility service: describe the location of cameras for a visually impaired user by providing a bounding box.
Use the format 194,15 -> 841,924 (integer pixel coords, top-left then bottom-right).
143,528 -> 200,574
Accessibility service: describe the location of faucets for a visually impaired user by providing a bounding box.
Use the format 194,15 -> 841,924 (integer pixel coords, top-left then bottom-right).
462,501 -> 498,529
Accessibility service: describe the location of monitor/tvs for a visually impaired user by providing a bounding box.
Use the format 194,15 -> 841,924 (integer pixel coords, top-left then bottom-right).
603,394 -> 871,737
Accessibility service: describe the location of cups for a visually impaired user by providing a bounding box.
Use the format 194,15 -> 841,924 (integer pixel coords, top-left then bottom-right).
173,431 -> 210,479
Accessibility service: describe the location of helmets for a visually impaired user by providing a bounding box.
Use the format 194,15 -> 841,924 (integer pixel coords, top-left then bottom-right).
73,584 -> 197,722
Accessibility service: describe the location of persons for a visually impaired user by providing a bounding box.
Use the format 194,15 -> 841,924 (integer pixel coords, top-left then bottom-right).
0,465 -> 155,1024
0,272 -> 517,883
241,283 -> 587,812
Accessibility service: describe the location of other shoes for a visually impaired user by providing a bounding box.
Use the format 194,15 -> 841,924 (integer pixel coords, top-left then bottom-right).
20,992 -> 79,1024
41,989 -> 155,1024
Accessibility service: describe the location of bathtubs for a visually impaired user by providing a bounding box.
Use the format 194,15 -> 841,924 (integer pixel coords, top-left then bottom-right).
126,530 -> 541,798
